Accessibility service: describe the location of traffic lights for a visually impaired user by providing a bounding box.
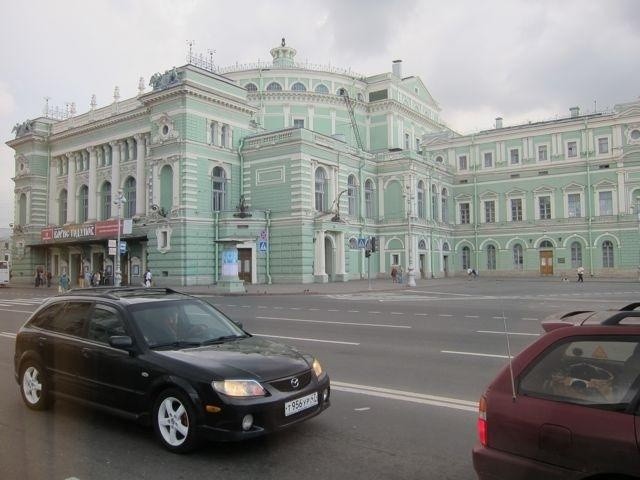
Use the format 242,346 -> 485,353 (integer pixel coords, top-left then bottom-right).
365,250 -> 372,257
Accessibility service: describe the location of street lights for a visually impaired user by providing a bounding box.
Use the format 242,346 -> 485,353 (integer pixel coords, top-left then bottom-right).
114,188 -> 126,289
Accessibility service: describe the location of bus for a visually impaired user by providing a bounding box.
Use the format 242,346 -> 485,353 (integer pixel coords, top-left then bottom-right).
0,261 -> 10,287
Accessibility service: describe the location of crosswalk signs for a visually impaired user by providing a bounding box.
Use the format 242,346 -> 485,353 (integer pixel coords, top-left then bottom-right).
358,238 -> 365,248
259,241 -> 267,251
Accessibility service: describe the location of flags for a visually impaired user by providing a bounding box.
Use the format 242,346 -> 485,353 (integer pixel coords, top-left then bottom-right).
122,217 -> 133,234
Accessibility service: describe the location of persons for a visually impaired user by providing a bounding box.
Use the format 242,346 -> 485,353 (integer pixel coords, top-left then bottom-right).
467,266 -> 476,281
577,265 -> 584,285
158,306 -> 202,344
33,268 -> 153,295
391,265 -> 403,283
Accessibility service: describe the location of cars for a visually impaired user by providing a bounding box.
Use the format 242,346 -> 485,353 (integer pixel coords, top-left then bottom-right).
14,287 -> 331,455
473,301 -> 639,479
